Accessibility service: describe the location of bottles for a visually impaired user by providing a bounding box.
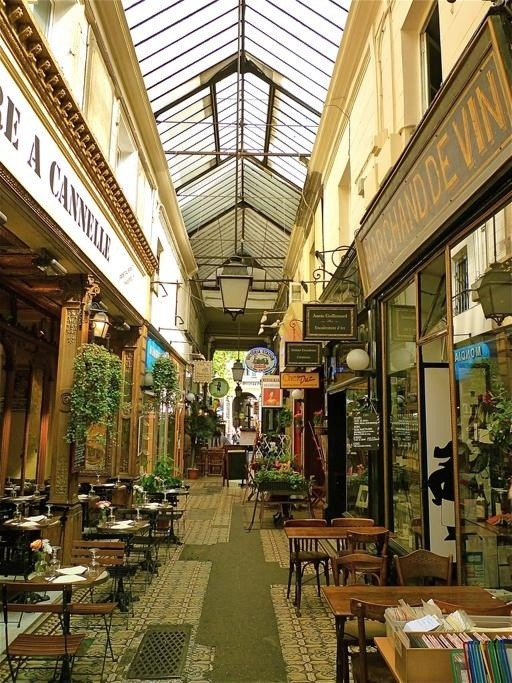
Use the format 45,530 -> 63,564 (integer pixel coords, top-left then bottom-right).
495,475 -> 507,526
475,482 -> 489,522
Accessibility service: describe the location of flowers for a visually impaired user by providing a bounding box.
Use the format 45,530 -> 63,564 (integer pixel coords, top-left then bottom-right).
253,458 -> 317,490
293,411 -> 304,425
312,409 -> 324,425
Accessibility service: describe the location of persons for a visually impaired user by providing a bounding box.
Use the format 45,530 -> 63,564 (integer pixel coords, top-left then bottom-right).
232,426 -> 242,446
213,430 -> 231,449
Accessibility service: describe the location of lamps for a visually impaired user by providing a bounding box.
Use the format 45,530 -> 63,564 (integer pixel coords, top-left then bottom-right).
214,0 -> 253,321
231,322 -> 245,381
346,349 -> 377,378
234,381 -> 243,397
84,307 -> 112,344
475,261 -> 512,326
290,389 -> 304,403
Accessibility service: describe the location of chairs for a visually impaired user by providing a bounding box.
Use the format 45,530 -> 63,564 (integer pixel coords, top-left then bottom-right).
221,445 -> 250,488
280,517 -> 512,683
0,477 -> 191,683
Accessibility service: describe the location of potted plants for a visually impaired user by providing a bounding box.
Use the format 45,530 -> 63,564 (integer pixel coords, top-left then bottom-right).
183,412 -> 217,479
140,452 -> 179,528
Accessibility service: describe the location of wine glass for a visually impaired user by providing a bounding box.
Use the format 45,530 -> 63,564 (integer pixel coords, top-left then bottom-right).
87,472 -> 169,506
12,502 -> 22,521
45,503 -> 54,521
86,548 -> 102,577
134,508 -> 143,526
49,545 -> 63,574
6,475 -> 41,500
106,506 -> 116,524
